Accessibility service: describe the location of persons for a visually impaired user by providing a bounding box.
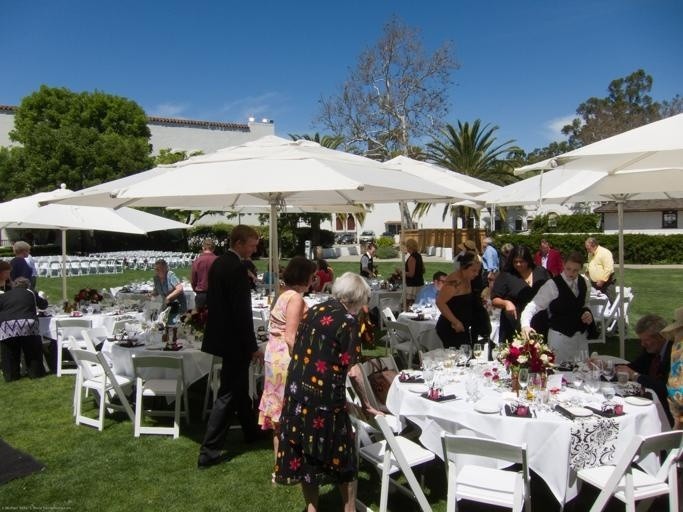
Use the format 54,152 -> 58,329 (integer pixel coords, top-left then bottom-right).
151,225 -> 261,467
272,272 -> 389,512
359,237 -> 683,428
2,240 -> 46,381
258,260 -> 336,479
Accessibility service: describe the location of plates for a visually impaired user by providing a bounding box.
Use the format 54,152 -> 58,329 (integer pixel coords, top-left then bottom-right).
625,397 -> 652,405
565,407 -> 592,418
473,400 -> 501,413
407,385 -> 428,393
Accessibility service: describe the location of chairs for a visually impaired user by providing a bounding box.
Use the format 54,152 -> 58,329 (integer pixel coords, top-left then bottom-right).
0,250 -> 201,277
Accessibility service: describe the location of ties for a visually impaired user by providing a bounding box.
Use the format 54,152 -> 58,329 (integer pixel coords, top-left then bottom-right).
570,279 -> 579,298
649,355 -> 660,377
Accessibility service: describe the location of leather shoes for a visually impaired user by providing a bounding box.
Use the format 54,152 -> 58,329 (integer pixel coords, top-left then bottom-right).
198,455 -> 230,470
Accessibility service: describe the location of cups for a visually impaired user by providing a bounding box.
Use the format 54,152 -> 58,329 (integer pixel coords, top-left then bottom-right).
616,372 -> 628,389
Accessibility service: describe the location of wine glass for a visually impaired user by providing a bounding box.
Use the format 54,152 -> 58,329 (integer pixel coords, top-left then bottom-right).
473,343 -> 482,375
460,344 -> 471,374
571,375 -> 582,392
491,350 -> 500,366
421,345 -> 457,400
576,350 -> 588,377
518,369 -> 528,396
602,360 -> 615,388
526,374 -> 540,405
601,385 -> 616,407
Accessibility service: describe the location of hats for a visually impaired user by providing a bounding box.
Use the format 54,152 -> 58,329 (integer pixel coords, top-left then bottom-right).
659,306 -> 683,341
458,240 -> 478,255
